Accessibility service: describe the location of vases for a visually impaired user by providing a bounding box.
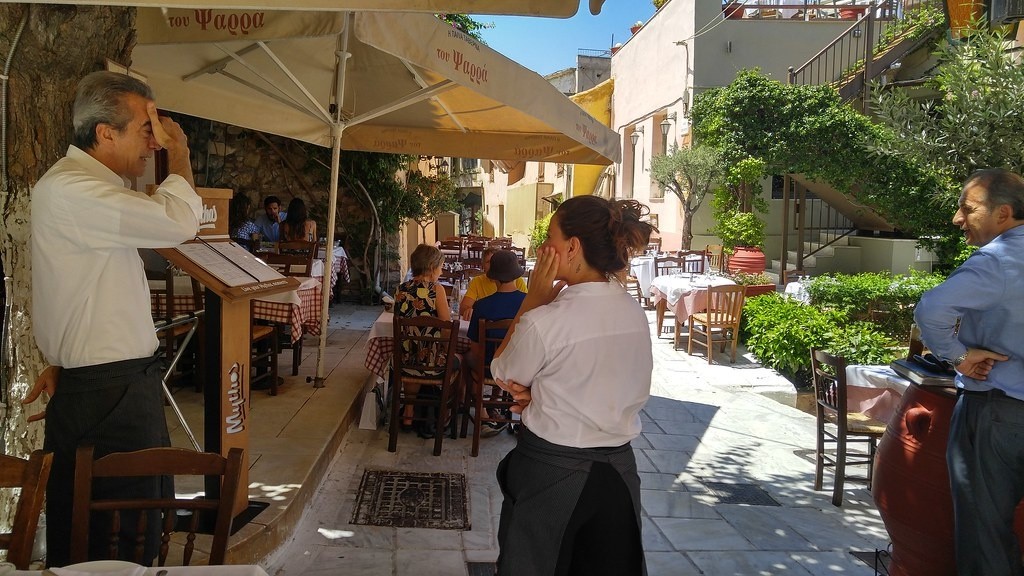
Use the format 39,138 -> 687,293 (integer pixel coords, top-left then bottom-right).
611,47 -> 620,54
723,3 -> 745,18
839,7 -> 865,19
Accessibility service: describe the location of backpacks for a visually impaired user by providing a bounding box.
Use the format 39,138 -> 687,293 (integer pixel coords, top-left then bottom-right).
414,384 -> 455,439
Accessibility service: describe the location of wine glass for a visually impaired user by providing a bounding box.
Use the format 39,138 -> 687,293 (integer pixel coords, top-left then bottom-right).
645,250 -> 650,257
443,261 -> 463,273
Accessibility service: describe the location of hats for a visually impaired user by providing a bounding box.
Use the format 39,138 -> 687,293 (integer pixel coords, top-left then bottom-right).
485,250 -> 525,282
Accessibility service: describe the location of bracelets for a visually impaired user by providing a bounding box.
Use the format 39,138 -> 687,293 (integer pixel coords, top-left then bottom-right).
953,348 -> 969,366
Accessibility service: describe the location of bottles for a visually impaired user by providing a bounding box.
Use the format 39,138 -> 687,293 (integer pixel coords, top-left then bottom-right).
447,278 -> 469,321
319,237 -> 341,249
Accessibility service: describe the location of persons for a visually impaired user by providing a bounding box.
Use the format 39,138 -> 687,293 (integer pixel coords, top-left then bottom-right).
490,195 -> 659,576
394,244 -> 462,432
462,249 -> 528,437
278,198 -> 318,254
912,171 -> 1024,576
23,70 -> 204,570
459,247 -> 528,316
250,196 -> 287,241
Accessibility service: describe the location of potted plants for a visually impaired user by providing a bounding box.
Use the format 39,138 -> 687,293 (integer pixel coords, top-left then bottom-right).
630,21 -> 643,34
720,213 -> 776,299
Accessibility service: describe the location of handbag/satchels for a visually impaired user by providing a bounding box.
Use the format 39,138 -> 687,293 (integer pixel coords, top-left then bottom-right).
359,382 -> 384,430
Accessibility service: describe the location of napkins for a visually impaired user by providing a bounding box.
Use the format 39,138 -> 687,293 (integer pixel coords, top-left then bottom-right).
48,567 -> 147,576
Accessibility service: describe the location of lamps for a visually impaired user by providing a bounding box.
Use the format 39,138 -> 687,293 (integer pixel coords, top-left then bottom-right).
630,126 -> 644,153
660,111 -> 677,145
853,30 -> 862,38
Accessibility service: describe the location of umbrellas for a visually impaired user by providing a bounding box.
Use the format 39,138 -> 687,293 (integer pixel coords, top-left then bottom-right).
0,0 -> 608,19
126,13 -> 621,388
590,166 -> 615,200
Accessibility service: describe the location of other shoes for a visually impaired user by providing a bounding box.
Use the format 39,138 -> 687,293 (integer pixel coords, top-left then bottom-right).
481,419 -> 510,435
445,408 -> 452,428
485,407 -> 500,417
400,414 -> 414,431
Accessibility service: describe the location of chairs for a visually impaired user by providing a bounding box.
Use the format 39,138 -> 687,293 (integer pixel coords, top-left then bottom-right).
148,233 -> 349,407
0,449 -> 55,571
808,346 -> 888,507
69,445 -> 244,568
387,234 -> 532,457
622,237 -> 748,365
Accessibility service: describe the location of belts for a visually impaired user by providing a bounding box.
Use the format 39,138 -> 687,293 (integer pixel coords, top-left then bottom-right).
964,388 -> 1007,398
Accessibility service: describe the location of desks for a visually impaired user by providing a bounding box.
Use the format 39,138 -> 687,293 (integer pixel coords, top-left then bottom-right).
148,240 -> 351,389
4,564 -> 271,576
649,274 -> 747,351
363,241 -> 530,410
824,365 -> 912,425
627,249 -> 711,311
